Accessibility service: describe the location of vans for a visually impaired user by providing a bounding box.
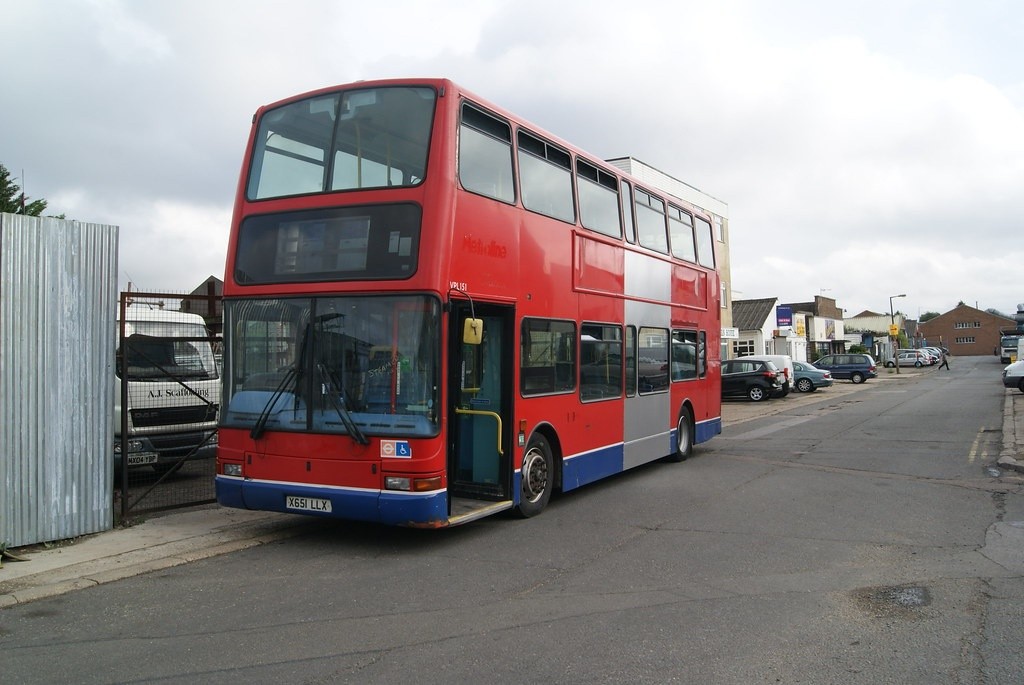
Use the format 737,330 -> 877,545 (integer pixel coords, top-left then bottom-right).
812,354 -> 878,384
720,355 -> 796,399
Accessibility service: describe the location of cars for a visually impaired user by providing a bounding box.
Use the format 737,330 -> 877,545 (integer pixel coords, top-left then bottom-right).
884,353 -> 930,369
1002,358 -> 1024,393
897,347 -> 942,364
792,361 -> 833,393
700,360 -> 782,401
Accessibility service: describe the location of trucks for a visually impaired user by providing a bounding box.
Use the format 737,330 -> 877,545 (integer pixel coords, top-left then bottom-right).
1000,335 -> 1021,364
116,307 -> 222,472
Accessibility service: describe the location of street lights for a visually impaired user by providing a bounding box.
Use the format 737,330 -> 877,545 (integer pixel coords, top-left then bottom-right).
890,294 -> 906,374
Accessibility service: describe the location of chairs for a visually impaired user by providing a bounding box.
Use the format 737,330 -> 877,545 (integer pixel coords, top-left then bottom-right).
469,182 -> 553,216
723,367 -> 727,374
580,381 -> 653,401
362,346 -> 411,417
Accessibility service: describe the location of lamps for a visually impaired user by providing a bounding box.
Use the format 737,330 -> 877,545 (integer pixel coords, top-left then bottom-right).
842,308 -> 847,312
772,336 -> 776,339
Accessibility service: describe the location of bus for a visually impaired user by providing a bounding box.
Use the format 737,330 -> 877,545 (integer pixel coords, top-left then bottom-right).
215,78 -> 722,528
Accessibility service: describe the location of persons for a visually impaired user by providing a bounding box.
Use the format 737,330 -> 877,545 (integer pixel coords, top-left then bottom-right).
994,345 -> 999,356
938,350 -> 951,370
